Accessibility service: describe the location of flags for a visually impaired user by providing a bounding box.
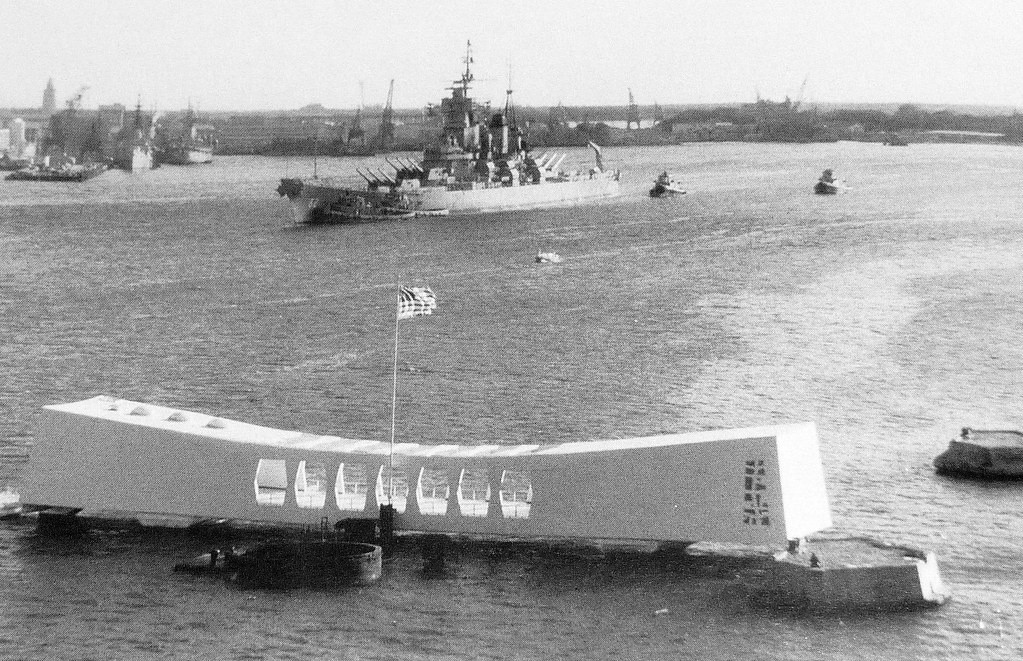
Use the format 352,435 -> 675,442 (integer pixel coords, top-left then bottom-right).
397,283 -> 438,320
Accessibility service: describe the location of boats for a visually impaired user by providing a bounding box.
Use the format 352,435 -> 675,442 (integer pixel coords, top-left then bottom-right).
111,93 -> 155,173
933,424 -> 1022,478
271,37 -> 624,227
164,97 -> 212,165
17,390 -> 953,614
889,133 -> 909,146
812,167 -> 855,196
647,168 -> 689,199
